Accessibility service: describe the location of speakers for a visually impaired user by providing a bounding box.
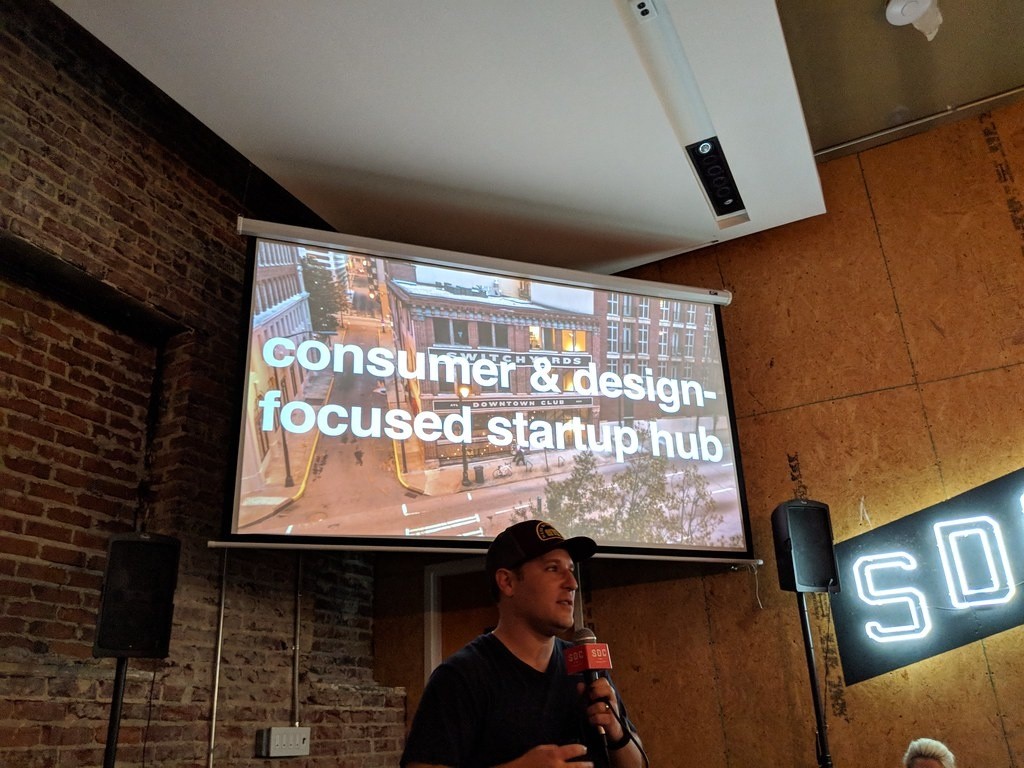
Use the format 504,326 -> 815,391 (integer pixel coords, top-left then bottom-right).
771,498 -> 841,593
92,532 -> 181,661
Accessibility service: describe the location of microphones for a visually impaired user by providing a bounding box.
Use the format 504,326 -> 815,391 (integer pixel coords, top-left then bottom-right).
563,628 -> 613,745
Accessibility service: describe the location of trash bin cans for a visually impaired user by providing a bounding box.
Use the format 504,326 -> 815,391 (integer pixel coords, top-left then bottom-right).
474,466 -> 484,483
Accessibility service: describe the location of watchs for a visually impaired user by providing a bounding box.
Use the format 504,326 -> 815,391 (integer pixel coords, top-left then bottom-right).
610,716 -> 630,749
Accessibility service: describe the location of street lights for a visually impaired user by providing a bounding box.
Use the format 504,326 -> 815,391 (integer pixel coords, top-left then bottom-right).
367,284 -> 385,334
457,385 -> 475,487
272,330 -> 340,487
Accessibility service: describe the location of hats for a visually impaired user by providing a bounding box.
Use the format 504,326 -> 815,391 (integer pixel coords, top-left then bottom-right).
486,520 -> 597,585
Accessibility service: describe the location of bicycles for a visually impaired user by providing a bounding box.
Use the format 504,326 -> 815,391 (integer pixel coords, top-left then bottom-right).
493,462 -> 514,479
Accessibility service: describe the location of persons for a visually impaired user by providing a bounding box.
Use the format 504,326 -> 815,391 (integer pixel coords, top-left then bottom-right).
397,519 -> 646,768
903,738 -> 955,768
